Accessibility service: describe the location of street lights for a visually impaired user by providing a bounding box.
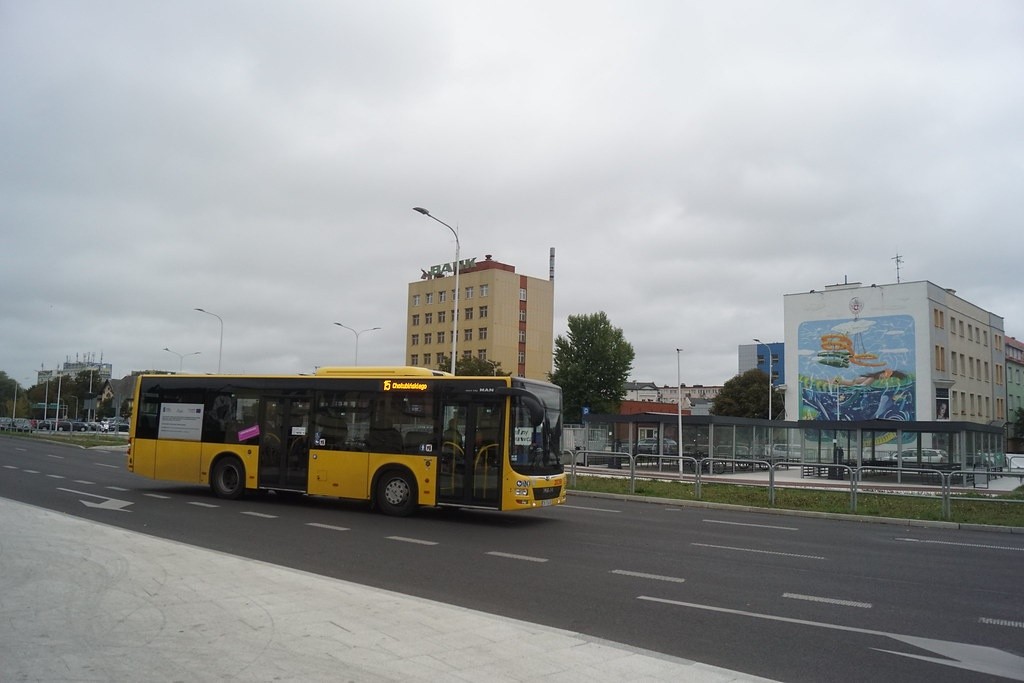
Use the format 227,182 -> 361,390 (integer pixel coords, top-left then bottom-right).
79,361 -> 102,422
333,321 -> 382,366
412,206 -> 461,376
32,369 -> 49,420
193,307 -> 224,374
163,347 -> 202,371
753,338 -> 773,443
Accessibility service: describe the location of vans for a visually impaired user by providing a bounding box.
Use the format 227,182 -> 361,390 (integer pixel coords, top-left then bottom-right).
772,444 -> 802,459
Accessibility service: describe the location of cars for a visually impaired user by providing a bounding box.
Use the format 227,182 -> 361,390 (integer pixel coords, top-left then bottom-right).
634,438 -> 676,452
105,418 -> 129,431
0,418 -> 98,431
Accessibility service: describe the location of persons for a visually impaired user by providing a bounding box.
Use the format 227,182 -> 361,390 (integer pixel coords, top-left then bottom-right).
937,402 -> 948,420
443,418 -> 463,470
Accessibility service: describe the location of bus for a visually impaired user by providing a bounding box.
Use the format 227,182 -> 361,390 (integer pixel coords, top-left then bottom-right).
127,366 -> 567,519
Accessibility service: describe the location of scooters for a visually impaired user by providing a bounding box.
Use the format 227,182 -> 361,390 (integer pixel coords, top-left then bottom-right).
98,420 -> 109,433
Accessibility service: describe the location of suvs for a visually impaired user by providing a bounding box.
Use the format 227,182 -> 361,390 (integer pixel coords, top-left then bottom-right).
882,448 -> 949,464
